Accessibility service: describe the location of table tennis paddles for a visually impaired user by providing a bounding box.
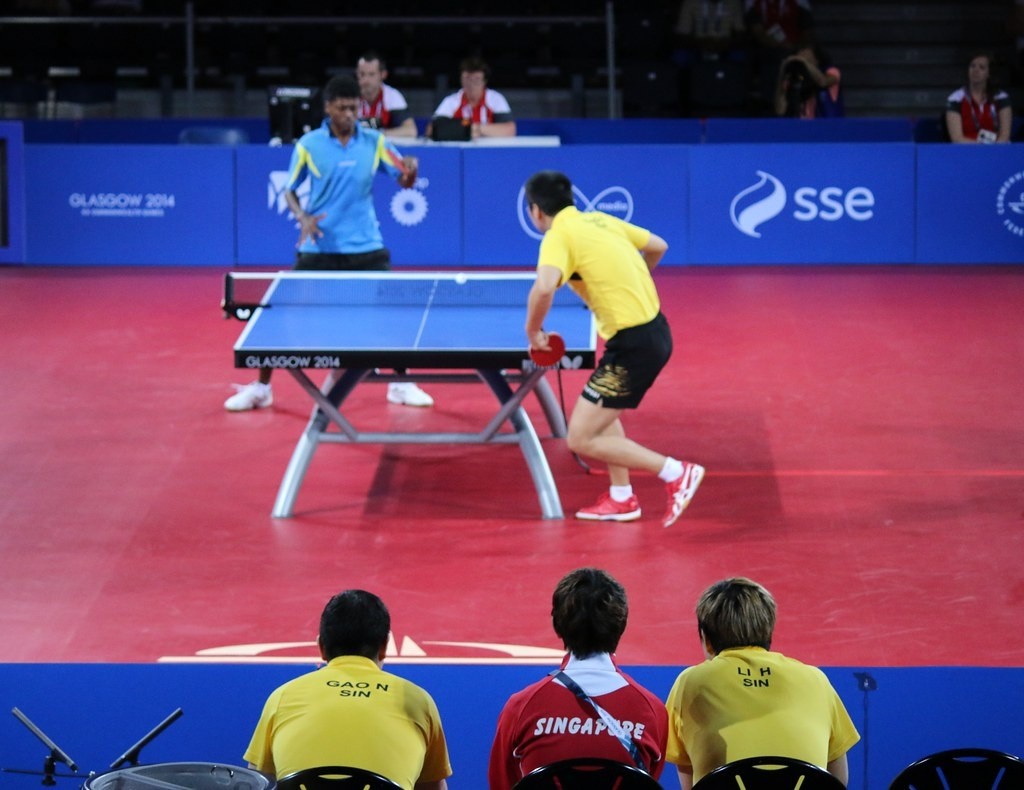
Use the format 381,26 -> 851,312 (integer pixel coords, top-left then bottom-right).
386,148 -> 409,176
528,326 -> 566,367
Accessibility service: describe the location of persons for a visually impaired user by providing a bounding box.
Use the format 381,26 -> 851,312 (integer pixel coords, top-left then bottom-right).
525,171 -> 705,528
665,576 -> 861,790
488,566 -> 669,790
946,49 -> 1012,144
424,55 -> 516,137
355,51 -> 418,137
776,44 -> 841,117
242,589 -> 453,790
224,76 -> 434,411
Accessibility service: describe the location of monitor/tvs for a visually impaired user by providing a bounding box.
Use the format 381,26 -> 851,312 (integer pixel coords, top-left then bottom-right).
266,83 -> 326,145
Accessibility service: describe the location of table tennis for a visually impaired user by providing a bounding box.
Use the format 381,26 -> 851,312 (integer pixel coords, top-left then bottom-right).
455,272 -> 467,284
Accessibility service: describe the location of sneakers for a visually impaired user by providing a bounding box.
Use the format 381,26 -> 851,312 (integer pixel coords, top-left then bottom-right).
661,459 -> 705,528
223,380 -> 273,411
575,491 -> 642,522
386,381 -> 434,407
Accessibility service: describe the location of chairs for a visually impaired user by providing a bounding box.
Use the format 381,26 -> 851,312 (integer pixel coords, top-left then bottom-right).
268,766 -> 404,790
888,748 -> 1024,790
691,756 -> 846,790
512,756 -> 664,790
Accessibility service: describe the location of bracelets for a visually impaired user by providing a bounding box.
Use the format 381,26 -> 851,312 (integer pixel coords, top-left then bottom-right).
294,209 -> 307,221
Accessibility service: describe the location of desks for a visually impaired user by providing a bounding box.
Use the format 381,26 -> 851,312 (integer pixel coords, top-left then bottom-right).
222,269 -> 597,520
0,664 -> 1024,790
178,125 -> 246,145
